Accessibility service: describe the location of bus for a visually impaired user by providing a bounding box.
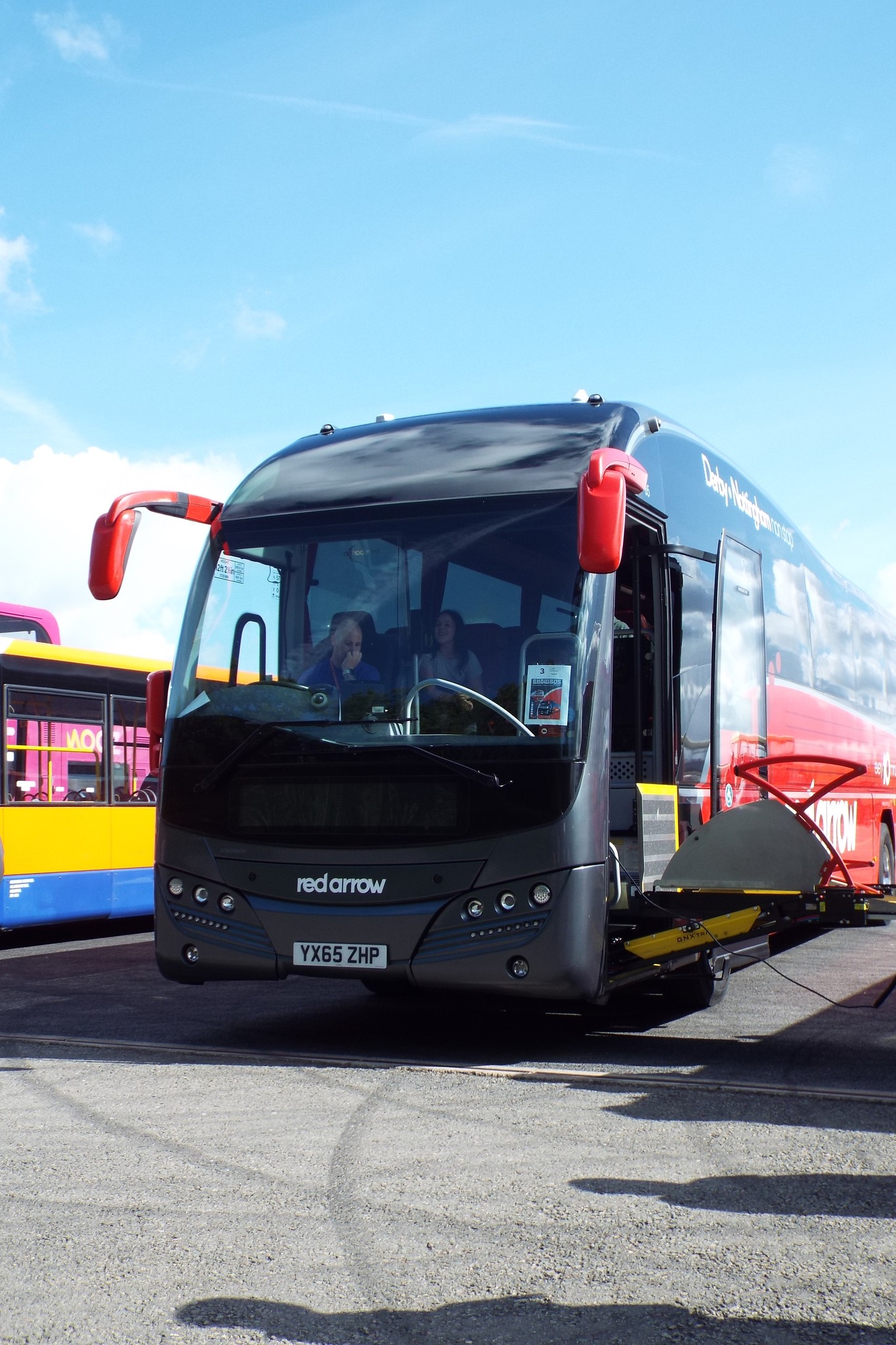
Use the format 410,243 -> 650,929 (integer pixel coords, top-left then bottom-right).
89,388 -> 894,1026
0,598 -> 278,933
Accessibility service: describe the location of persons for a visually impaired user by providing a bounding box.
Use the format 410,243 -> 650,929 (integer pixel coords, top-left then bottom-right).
295,614 -> 383,700
138,772 -> 158,801
414,609 -> 485,711
566,613 -> 635,639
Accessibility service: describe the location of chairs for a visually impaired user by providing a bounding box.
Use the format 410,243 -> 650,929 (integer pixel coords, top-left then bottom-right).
8,787 -> 157,802
313,611 -> 541,697
